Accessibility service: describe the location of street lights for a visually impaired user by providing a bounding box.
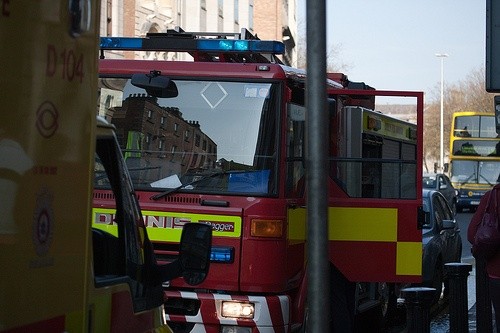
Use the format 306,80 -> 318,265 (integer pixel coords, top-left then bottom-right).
435,53 -> 448,174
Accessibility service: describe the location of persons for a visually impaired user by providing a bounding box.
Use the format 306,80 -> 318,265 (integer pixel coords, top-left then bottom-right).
467,173 -> 500,332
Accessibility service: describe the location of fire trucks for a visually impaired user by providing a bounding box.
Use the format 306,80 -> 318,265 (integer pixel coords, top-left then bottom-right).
91,26 -> 423,333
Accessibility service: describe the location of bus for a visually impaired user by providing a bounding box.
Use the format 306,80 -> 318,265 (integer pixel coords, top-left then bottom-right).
448,112 -> 500,213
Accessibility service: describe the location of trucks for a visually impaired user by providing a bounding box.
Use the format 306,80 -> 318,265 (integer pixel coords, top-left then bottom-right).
0,0 -> 212,333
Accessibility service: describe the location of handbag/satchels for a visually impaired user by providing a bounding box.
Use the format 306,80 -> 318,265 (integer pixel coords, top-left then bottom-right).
471,186 -> 500,262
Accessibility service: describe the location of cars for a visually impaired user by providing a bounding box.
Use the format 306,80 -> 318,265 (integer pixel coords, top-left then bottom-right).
396,174 -> 462,313
